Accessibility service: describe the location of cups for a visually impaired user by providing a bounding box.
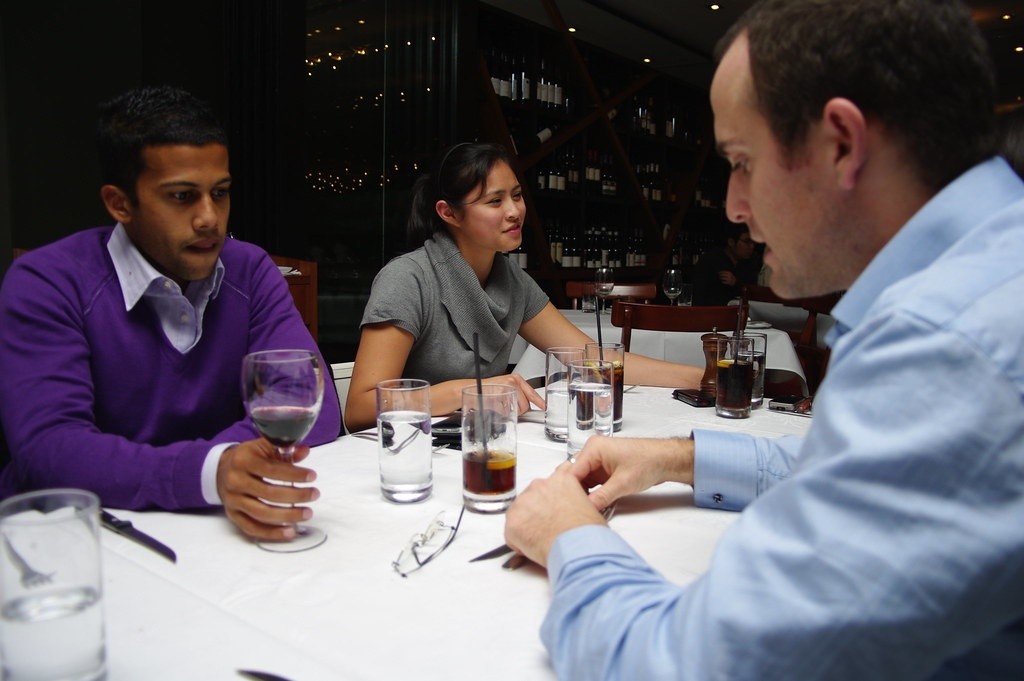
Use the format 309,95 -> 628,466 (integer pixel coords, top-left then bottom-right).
731,333 -> 767,409
565,359 -> 614,469
583,343 -> 624,432
571,363 -> 596,430
460,382 -> 517,514
716,337 -> 753,418
544,346 -> 590,443
676,281 -> 694,307
0,486 -> 112,681
582,283 -> 597,314
376,378 -> 433,504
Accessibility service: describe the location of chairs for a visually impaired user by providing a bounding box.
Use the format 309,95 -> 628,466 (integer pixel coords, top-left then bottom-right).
612,283 -> 831,394
566,281 -> 657,309
15,247 -> 320,348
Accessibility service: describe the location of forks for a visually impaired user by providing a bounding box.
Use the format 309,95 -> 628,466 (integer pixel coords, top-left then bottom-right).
503,497 -> 618,572
356,432 -> 451,453
0,529 -> 53,590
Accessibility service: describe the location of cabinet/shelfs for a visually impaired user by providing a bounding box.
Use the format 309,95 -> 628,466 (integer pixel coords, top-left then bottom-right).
479,0 -> 743,302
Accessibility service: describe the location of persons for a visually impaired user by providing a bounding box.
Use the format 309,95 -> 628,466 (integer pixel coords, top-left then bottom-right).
0,86 -> 341,541
695,224 -> 757,306
345,142 -> 705,434
503,0 -> 1024,681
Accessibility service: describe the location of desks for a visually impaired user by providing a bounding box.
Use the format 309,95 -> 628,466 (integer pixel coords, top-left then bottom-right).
511,307 -> 813,396
725,299 -> 832,348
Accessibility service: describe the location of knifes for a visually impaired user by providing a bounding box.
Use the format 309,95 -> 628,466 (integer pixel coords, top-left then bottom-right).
467,541 -> 511,563
76,499 -> 177,563
235,669 -> 294,680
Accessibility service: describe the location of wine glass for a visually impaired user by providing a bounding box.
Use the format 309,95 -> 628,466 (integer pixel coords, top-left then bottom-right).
593,267 -> 615,315
661,269 -> 683,308
240,348 -> 328,553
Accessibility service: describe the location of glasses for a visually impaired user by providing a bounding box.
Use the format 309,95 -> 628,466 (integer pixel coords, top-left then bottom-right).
394,499 -> 466,579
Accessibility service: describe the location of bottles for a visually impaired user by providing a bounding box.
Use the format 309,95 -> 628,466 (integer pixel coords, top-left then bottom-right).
456,13 -> 754,279
700,327 -> 728,397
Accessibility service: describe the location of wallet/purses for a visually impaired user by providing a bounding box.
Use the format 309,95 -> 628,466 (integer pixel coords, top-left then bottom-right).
431,409 -> 499,451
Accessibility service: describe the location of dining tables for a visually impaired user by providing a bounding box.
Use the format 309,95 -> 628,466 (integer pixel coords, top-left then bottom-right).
0,378 -> 810,681
